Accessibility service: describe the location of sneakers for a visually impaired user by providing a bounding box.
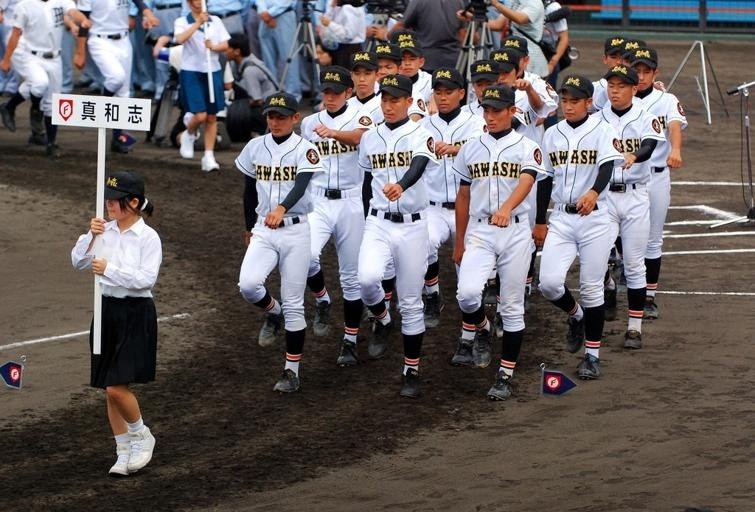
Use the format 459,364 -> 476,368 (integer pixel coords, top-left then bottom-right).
130,430 -> 156,473
257,290 -> 446,398
199,156 -> 219,172
451,278 -> 659,401
178,131 -> 194,157
109,446 -> 128,477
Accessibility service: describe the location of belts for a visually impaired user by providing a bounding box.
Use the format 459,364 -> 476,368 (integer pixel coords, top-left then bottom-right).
278,214 -> 300,229
429,199 -> 456,212
608,182 -> 637,194
476,214 -> 521,227
323,187 -> 342,201
97,28 -> 131,41
28,49 -> 62,60
154,3 -> 181,10
210,9 -> 243,19
370,208 -> 421,224
561,202 -> 600,216
652,166 -> 666,175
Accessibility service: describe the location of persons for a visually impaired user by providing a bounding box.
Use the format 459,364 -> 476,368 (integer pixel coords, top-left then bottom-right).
585,65 -> 663,354
0,0 -> 68,159
72,0 -> 161,154
533,75 -> 619,381
232,89 -> 321,393
1,0 -> 572,153
449,82 -> 541,397
69,166 -> 164,478
352,73 -> 439,399
300,66 -> 375,369
167,1 -> 229,174
233,32 -> 689,372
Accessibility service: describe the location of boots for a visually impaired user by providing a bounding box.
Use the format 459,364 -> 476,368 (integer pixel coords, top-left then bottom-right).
45,115 -> 66,159
29,93 -> 46,145
0,90 -> 25,132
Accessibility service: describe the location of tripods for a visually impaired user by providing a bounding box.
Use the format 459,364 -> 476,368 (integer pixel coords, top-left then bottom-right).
664,39 -> 730,126
453,13 -> 494,81
365,13 -> 391,52
276,15 -> 326,92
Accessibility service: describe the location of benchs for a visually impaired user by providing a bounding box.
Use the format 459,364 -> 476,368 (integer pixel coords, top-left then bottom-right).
591,1 -> 755,32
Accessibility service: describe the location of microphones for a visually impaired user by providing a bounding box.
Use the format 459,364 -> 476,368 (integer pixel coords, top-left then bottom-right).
726,81 -> 755,95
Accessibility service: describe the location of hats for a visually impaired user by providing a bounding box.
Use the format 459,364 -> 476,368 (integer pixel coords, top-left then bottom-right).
102,173 -> 145,199
320,30 -> 658,110
262,94 -> 299,118
